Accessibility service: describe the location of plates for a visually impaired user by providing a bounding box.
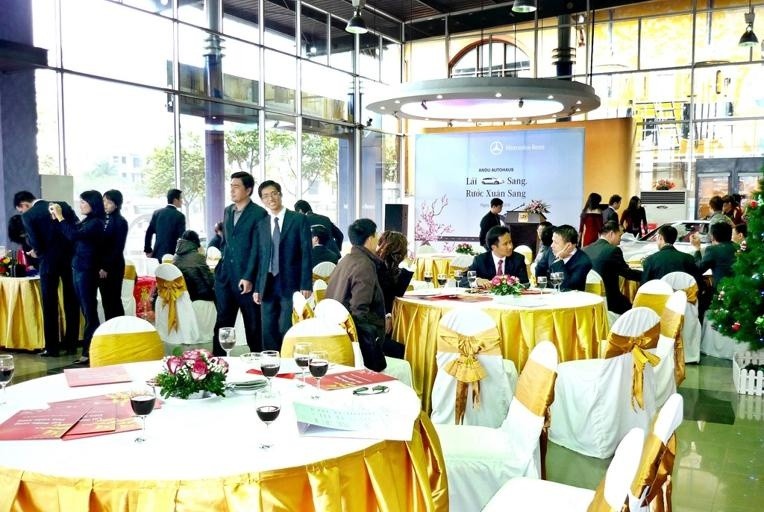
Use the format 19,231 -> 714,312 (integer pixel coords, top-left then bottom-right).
221,374 -> 265,392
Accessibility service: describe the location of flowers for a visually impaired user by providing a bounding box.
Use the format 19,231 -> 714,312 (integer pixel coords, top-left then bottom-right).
524,199 -> 552,216
411,194 -> 458,245
651,177 -> 676,192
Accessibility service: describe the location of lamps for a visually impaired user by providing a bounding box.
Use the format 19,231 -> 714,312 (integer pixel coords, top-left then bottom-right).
343,0 -> 372,35
515,98 -> 523,108
734,0 -> 760,48
421,100 -> 429,110
447,119 -> 452,127
567,105 -> 577,117
510,0 -> 538,15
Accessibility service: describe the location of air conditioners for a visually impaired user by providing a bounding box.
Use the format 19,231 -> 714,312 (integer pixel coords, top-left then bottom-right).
637,190 -> 690,228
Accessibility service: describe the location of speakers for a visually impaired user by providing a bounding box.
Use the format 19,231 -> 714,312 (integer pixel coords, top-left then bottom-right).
385,204 -> 408,237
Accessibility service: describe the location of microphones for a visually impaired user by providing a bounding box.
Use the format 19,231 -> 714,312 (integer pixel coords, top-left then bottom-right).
511,202 -> 526,211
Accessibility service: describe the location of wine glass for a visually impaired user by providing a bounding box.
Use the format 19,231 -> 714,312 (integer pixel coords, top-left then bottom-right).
218,326 -> 237,363
550,271 -> 565,295
308,350 -> 331,399
536,276 -> 548,296
260,349 -> 282,398
293,342 -> 313,390
1,355 -> 16,405
425,267 -> 476,296
252,385 -> 283,454
124,379 -> 155,445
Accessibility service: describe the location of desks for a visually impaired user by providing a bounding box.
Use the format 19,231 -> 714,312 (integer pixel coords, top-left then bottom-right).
1,356 -> 449,512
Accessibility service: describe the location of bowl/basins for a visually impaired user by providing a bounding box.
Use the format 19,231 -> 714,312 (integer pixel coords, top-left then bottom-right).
240,352 -> 263,365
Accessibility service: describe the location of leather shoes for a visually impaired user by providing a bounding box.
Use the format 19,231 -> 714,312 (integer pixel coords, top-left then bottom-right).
73,358 -> 89,364
38,350 -> 60,357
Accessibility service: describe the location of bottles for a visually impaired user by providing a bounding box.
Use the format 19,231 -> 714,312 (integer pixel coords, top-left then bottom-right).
199,230 -> 208,256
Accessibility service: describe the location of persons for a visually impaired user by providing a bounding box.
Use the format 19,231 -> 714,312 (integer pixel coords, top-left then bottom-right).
456,198 -> 529,288
643,225 -> 704,288
8,190 -> 224,364
213,173 -> 268,356
530,219 -> 647,316
579,193 -> 648,249
253,181 -> 313,357
689,193 -> 747,294
296,199 -> 413,371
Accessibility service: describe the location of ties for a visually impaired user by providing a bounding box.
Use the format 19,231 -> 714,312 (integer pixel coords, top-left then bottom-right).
498,260 -> 503,276
271,218 -> 281,277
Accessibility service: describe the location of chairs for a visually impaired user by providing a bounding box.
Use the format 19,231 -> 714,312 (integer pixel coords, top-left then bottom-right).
425,339 -> 559,512
478,427 -> 646,512
627,392 -> 685,511
431,305 -> 518,429
157,237 -> 743,418
279,318 -> 356,369
157,261 -> 218,345
547,307 -> 662,458
0,238 -> 156,353
87,315 -> 165,367
